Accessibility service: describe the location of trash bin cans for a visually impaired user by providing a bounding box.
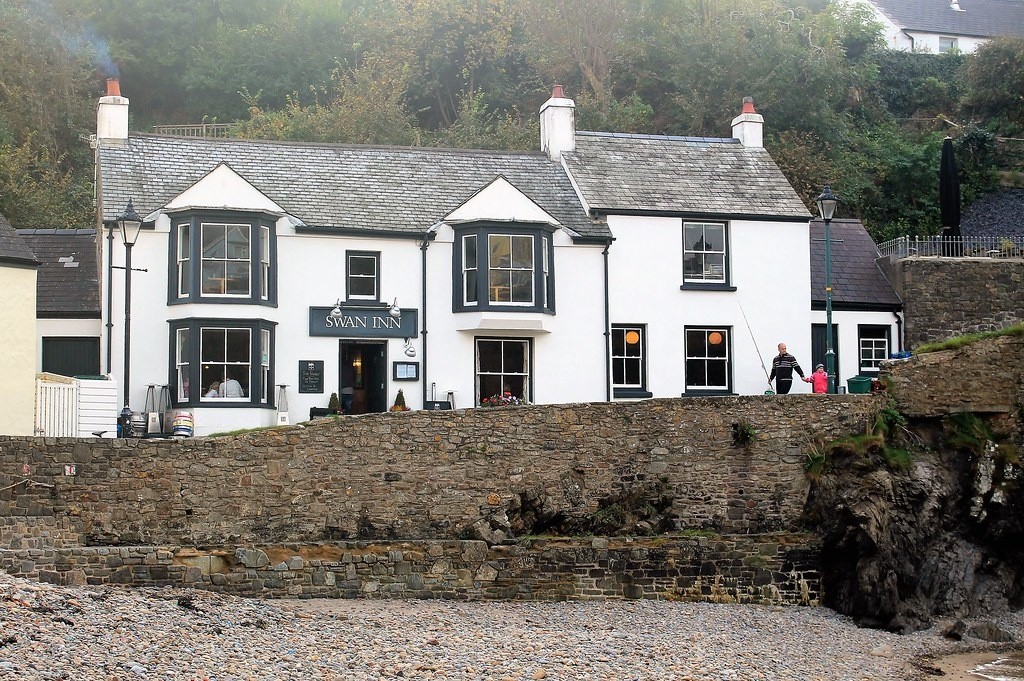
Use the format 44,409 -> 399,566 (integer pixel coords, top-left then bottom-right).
847,376 -> 872,394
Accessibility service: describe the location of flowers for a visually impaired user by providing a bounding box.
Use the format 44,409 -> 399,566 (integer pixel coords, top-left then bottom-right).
480,391 -> 521,405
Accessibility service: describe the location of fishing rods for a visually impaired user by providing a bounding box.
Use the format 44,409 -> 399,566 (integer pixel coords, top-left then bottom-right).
733,290 -> 776,392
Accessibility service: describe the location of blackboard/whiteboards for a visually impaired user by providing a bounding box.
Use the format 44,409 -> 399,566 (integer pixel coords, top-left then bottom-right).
299,360 -> 325,393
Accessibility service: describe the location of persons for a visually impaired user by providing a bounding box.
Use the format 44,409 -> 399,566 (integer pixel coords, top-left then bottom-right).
801,363 -> 828,393
203,382 -> 220,398
218,371 -> 244,398
768,343 -> 807,394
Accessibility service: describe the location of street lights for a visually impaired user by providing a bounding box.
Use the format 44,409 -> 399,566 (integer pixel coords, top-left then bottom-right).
816,183 -> 838,396
116,197 -> 144,435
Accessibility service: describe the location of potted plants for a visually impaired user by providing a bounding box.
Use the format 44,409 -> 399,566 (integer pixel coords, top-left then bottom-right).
328,393 -> 339,414
390,389 -> 409,411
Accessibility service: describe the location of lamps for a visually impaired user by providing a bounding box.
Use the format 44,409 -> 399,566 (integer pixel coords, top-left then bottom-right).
330,299 -> 342,317
389,297 -> 401,317
405,337 -> 416,357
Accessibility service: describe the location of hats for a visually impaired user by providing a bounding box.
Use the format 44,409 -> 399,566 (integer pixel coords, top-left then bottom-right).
816,361 -> 824,370
